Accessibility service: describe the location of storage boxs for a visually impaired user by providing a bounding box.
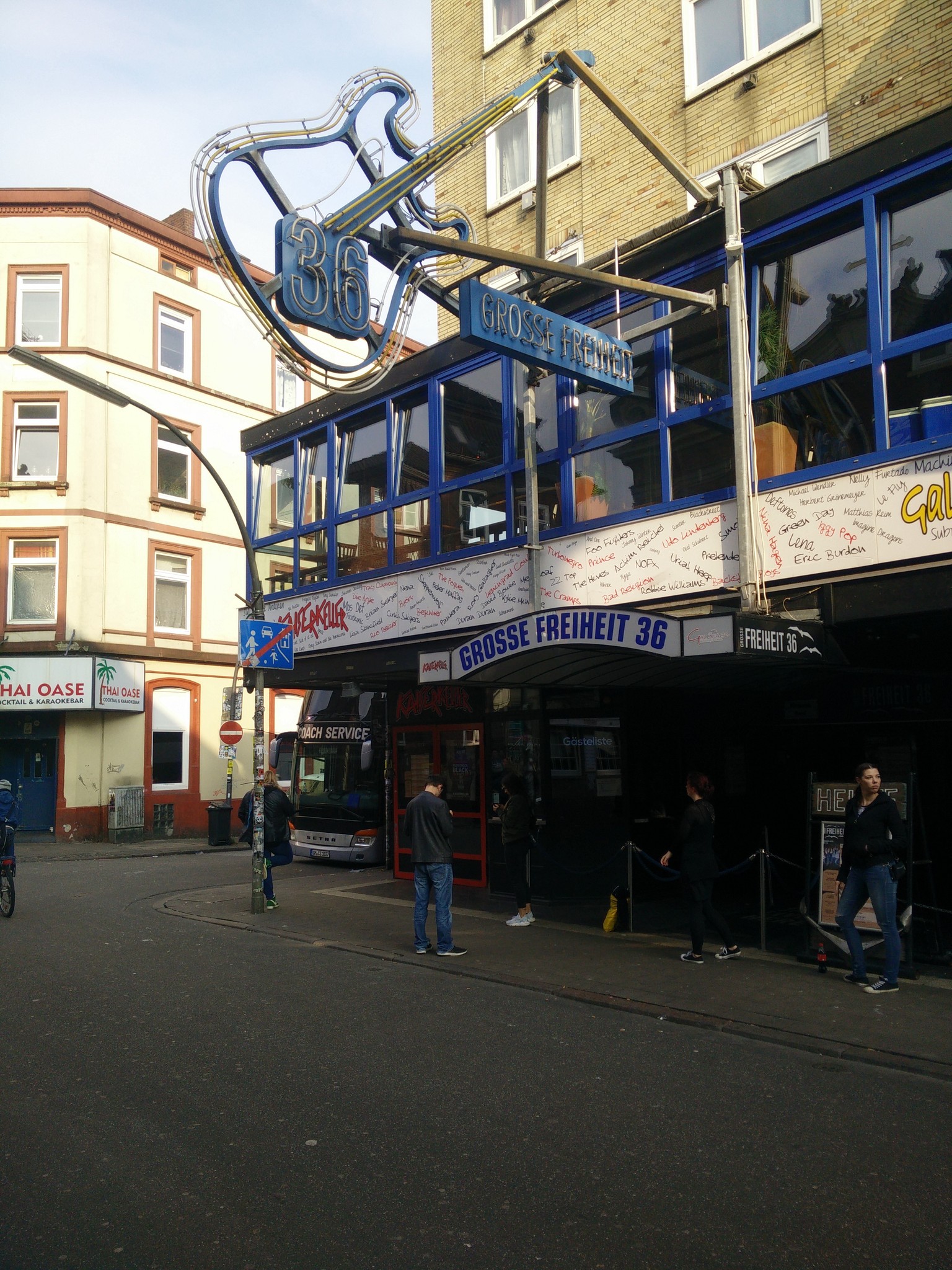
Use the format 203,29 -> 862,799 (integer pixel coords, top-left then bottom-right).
872,395 -> 952,451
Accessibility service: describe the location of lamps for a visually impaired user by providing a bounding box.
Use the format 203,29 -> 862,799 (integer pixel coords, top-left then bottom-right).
340,678 -> 364,698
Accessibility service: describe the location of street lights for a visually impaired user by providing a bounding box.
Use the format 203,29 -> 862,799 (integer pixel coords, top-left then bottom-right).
8,347 -> 271,915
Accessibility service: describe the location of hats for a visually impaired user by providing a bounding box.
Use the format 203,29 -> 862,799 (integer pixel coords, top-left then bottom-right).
0,780 -> 12,791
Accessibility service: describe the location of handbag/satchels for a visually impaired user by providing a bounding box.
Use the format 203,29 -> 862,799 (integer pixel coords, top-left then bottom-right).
604,894 -> 631,933
886,856 -> 905,880
0,821 -> 7,855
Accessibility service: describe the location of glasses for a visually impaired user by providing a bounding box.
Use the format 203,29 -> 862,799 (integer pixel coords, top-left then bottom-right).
501,786 -> 507,791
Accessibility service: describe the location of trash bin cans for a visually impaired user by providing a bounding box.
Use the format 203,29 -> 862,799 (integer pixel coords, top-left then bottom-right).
205,800 -> 234,845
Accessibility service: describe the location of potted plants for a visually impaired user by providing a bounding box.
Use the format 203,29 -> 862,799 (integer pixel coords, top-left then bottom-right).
748,304 -> 798,480
556,383 -> 612,522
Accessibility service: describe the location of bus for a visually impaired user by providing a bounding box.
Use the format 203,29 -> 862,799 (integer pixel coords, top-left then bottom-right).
268,706 -> 386,866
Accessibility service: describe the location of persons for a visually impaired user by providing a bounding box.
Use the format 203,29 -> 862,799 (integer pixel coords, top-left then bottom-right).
836,763 -> 910,993
238,771 -> 297,909
493,773 -> 536,926
403,775 -> 468,956
0,779 -> 22,858
661,771 -> 741,963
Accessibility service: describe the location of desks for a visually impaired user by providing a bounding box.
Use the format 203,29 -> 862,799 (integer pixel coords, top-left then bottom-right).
304,554 -> 342,563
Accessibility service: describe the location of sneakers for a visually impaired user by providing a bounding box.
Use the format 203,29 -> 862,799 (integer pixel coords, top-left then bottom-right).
506,912 -> 531,927
715,946 -> 741,960
843,972 -> 870,986
863,977 -> 900,994
267,894 -> 279,909
437,945 -> 468,955
680,950 -> 703,964
512,911 -> 536,923
417,943 -> 432,954
263,857 -> 272,880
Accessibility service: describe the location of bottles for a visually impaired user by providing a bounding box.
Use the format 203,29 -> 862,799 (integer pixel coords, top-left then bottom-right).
818,943 -> 827,974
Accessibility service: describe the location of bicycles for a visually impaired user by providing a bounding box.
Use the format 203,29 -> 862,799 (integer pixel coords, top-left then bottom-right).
0,851 -> 17,917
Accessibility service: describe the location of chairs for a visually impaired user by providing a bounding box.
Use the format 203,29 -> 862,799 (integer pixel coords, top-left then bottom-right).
338,542 -> 358,559
375,535 -> 388,551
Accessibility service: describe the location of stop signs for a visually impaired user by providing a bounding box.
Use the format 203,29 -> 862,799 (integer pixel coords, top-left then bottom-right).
219,721 -> 244,745
